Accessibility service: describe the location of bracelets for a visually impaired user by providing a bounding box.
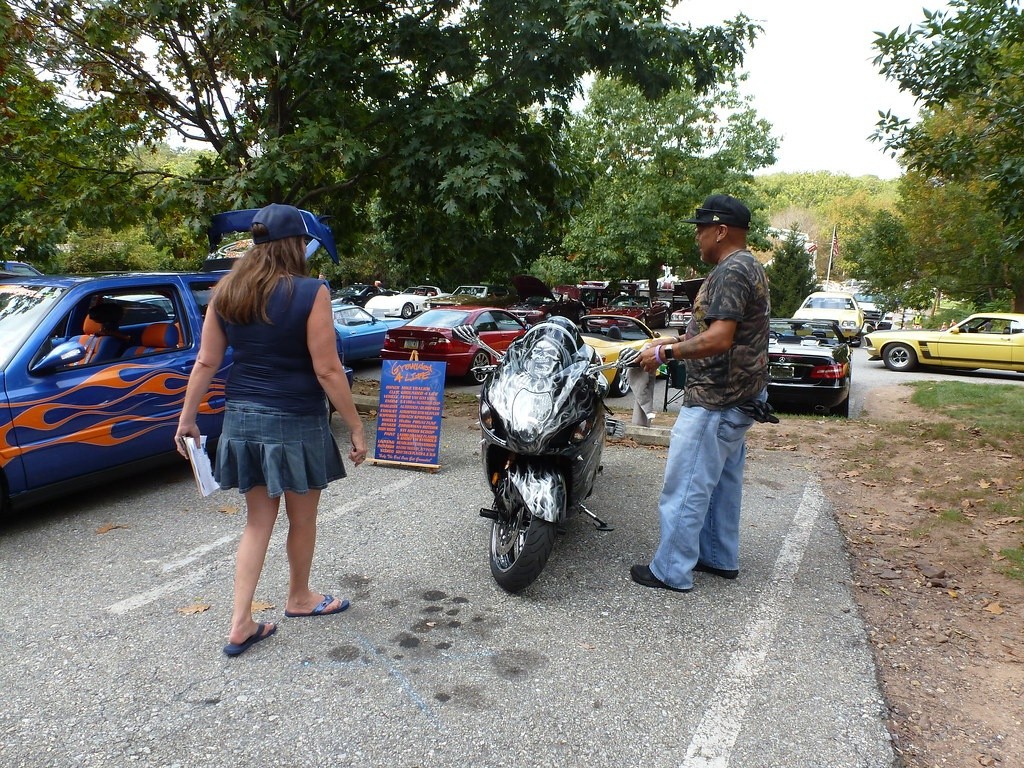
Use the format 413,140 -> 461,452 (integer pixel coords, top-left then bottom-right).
673,335 -> 680,343
654,345 -> 663,365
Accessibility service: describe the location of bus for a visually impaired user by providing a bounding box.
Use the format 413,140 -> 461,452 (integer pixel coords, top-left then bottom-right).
576,281 -> 640,308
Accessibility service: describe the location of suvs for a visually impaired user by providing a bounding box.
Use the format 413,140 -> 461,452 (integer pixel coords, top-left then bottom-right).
852,292 -> 894,330
420,286 -> 510,312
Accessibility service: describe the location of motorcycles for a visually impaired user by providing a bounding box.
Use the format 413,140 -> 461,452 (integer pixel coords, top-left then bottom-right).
450,313 -> 649,592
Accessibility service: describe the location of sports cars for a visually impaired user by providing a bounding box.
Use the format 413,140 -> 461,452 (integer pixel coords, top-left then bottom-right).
570,315 -> 669,396
365,285 -> 451,319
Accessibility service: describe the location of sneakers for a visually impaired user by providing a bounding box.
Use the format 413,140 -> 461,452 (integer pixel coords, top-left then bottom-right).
631,564 -> 693,592
692,562 -> 739,578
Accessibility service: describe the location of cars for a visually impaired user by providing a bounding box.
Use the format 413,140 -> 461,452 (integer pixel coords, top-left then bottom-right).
510,276 -> 580,324
635,278 -> 707,335
331,284 -> 381,308
863,311 -> 1024,381
0,206 -> 354,522
381,307 -> 533,387
578,296 -> 671,331
759,226 -> 871,292
767,317 -> 852,418
789,289 -> 866,347
331,306 -> 411,365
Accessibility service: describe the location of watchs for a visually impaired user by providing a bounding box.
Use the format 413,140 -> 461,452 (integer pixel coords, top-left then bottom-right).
665,343 -> 675,361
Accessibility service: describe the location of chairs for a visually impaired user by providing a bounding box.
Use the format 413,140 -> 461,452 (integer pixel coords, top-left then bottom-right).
769,333 -> 778,341
66,303 -> 123,366
606,325 -> 622,339
803,336 -> 821,343
1003,327 -> 1010,333
121,323 -> 179,357
663,359 -> 687,411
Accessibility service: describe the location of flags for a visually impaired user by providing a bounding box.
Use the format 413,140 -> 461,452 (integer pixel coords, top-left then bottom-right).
832,230 -> 839,255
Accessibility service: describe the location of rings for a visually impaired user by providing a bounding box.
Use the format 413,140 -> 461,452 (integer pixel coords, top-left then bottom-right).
361,456 -> 366,460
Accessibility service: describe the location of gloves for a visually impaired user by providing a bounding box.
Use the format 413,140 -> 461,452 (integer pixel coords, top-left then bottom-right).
737,399 -> 779,424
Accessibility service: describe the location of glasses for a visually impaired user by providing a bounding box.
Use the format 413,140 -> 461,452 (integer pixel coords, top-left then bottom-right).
696,208 -> 732,218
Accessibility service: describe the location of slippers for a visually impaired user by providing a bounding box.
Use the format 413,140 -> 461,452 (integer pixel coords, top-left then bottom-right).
284,595 -> 349,617
223,622 -> 276,654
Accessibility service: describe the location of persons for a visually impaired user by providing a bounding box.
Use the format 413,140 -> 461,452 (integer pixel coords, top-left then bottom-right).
175,202 -> 367,655
630,194 -> 769,593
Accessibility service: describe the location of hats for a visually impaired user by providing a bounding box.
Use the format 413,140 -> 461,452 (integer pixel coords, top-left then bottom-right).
251,203 -> 322,244
680,196 -> 750,230
